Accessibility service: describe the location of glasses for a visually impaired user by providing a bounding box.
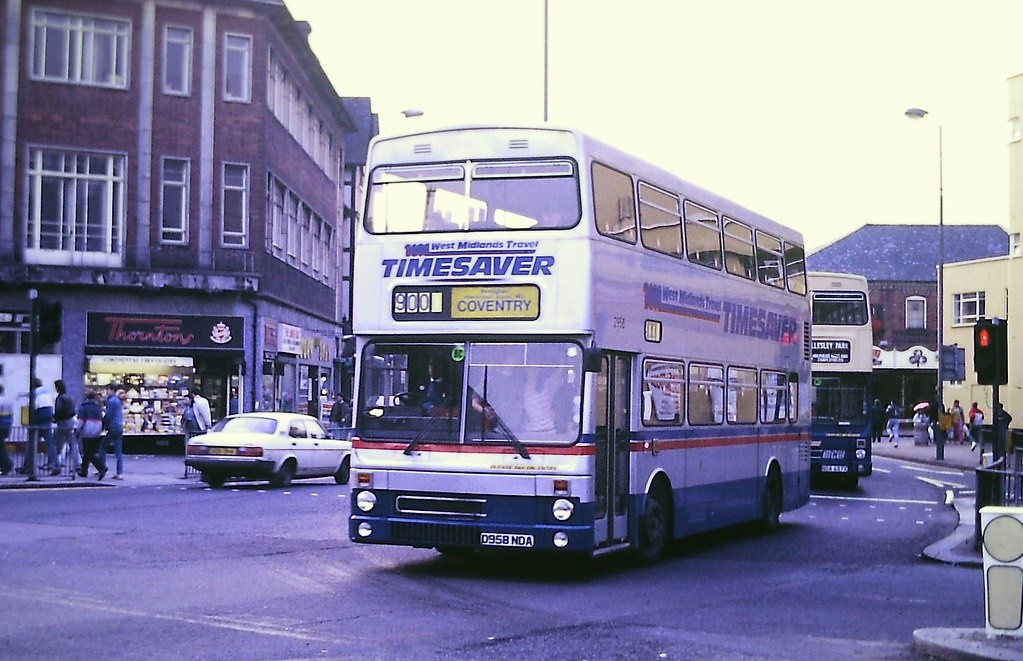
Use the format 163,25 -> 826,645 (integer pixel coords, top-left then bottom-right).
426,361 -> 446,368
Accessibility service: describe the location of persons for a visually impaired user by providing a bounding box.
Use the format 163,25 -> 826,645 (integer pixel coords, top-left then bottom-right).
471,354 -> 567,433
40,381 -> 83,471
180,388 -> 211,447
96,382 -> 123,479
329,393 -> 350,426
884,400 -> 904,448
871,399 -> 886,442
1000,403 -> 1013,429
968,402 -> 985,457
15,378 -> 62,475
75,392 -> 108,480
0,384 -> 15,476
913,399 -> 965,444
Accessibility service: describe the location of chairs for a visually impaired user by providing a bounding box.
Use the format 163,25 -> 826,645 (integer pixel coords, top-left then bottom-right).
598,221 -> 780,287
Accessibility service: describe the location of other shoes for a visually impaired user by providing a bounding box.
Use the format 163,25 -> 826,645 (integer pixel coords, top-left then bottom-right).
15,467 -> 31,474
889,435 -> 894,442
971,441 -> 977,451
79,471 -> 88,477
98,467 -> 109,481
70,467 -> 82,472
895,444 -> 898,448
39,463 -> 52,470
50,469 -> 61,476
0,465 -> 12,475
113,475 -> 123,480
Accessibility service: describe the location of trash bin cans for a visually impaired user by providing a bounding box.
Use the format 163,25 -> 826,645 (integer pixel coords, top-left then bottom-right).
914,423 -> 928,446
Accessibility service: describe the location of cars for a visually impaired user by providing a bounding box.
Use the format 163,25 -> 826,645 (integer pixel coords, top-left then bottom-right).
185,412 -> 352,487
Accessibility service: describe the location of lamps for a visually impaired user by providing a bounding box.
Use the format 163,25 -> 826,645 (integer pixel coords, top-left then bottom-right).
95,273 -> 105,285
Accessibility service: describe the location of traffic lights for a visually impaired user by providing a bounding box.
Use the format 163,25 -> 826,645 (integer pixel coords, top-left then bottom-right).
974,319 -> 1007,386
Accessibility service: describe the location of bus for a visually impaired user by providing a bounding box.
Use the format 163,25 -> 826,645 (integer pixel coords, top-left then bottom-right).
805,272 -> 873,483
348,124 -> 811,567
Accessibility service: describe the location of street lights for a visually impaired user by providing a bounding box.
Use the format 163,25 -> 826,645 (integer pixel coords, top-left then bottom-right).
905,109 -> 944,458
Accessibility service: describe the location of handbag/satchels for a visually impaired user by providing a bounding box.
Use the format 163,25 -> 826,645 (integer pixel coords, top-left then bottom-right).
962,424 -> 970,437
